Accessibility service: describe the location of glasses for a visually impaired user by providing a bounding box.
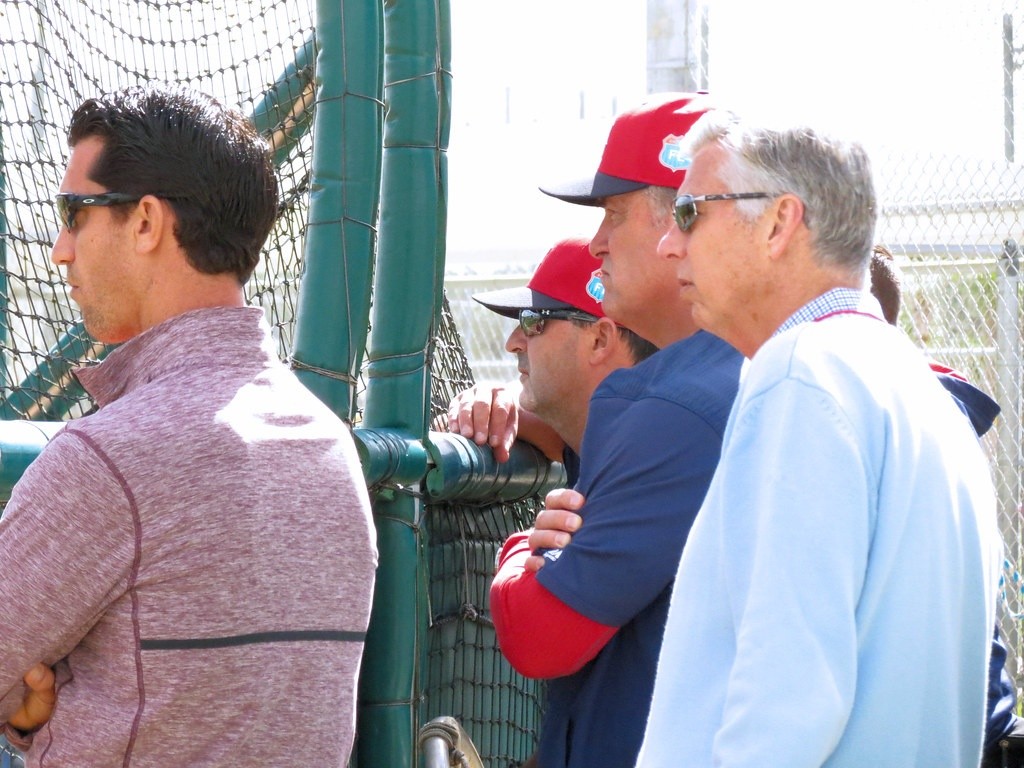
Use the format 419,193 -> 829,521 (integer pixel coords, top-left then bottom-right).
670,187 -> 771,232
518,305 -> 599,336
55,183 -> 186,234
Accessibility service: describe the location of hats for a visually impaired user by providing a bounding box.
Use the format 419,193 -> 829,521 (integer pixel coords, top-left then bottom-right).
471,239 -> 627,329
538,84 -> 718,207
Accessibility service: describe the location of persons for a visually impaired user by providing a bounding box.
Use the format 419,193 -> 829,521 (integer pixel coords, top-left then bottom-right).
1,83 -> 379,768
446,92 -> 1024,768
634,77 -> 1001,767
